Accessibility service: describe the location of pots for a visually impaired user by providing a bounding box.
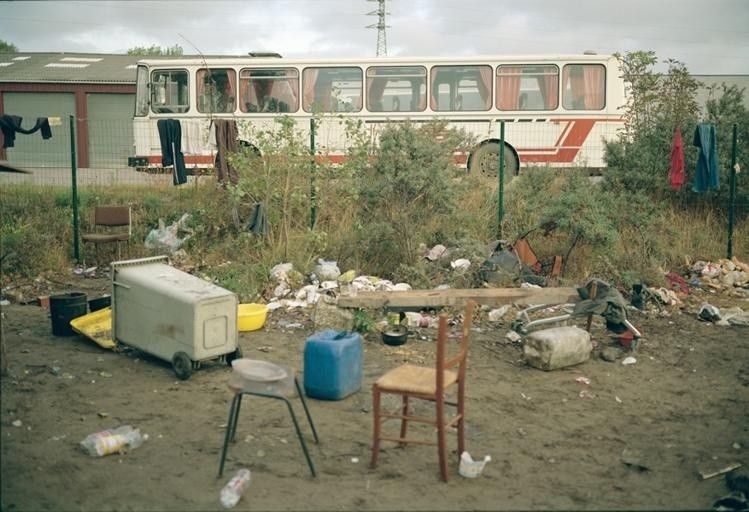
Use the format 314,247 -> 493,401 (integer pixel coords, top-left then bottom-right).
381,323 -> 408,346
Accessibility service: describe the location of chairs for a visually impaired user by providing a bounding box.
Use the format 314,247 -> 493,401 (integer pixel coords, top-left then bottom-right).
391,96 -> 400,112
80,207 -> 132,267
224,96 -> 234,112
456,95 -> 463,111
371,301 -> 475,484
260,95 -> 290,112
579,95 -> 585,110
518,93 -> 528,109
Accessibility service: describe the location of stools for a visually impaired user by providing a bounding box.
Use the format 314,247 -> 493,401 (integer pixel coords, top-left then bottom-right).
218,367 -> 319,477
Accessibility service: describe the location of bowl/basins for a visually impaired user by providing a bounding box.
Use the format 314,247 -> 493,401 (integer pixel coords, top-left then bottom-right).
238,303 -> 269,332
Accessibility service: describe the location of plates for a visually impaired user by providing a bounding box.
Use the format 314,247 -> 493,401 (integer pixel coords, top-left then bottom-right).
231,357 -> 289,383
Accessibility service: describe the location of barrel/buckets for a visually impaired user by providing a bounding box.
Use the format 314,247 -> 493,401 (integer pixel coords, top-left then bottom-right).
304,326 -> 363,399
89,295 -> 111,311
49,290 -> 86,337
520,322 -> 597,370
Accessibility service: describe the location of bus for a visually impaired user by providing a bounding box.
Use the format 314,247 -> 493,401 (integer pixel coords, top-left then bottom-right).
126,55 -> 634,187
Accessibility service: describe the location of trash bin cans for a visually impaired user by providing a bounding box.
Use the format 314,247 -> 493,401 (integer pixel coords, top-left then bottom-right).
69,255 -> 239,379
